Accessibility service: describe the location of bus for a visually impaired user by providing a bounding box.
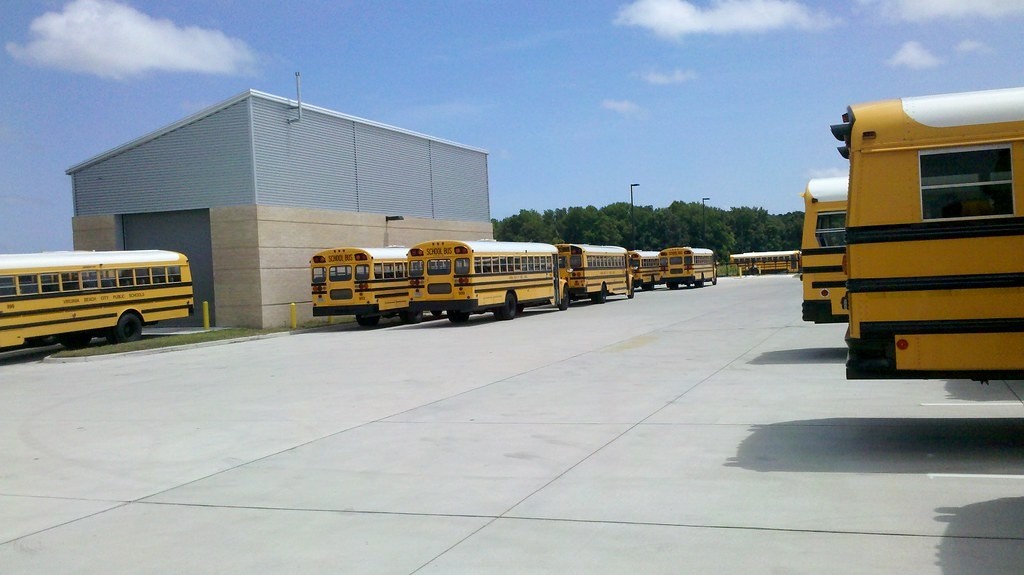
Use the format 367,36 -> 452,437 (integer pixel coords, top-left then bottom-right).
627,250 -> 666,292
406,240 -> 570,326
656,247 -> 717,291
553,244 -> 637,304
728,250 -> 801,275
310,248 -> 427,328
801,177 -> 852,325
834,86 -> 1024,379
0,250 -> 193,347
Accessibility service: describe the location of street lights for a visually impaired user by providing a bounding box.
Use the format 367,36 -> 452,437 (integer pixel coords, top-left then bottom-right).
630,183 -> 642,250
702,197 -> 710,248
